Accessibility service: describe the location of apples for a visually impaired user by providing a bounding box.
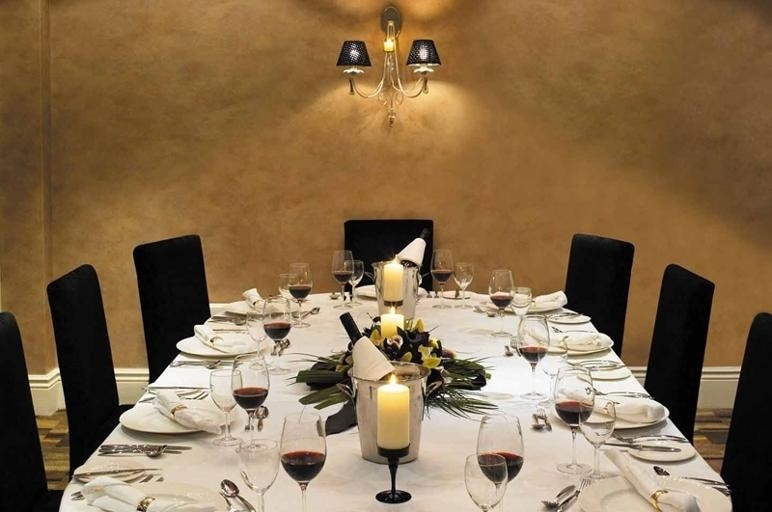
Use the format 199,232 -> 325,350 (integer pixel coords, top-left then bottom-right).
377,374 -> 410,449
382,262 -> 404,303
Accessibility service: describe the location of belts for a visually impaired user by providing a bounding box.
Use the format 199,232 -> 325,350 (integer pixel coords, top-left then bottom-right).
578,399 -> 616,481
478,412 -> 525,511
235,439 -> 280,511
280,410 -> 328,510
232,354 -> 277,452
464,454 -> 510,512
210,367 -> 245,446
554,363 -> 592,476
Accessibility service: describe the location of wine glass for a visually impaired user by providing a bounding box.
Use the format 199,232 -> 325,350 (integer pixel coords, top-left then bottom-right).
478,412 -> 525,511
264,296 -> 292,369
511,288 -> 532,348
464,454 -> 510,512
331,250 -> 355,310
280,410 -> 328,510
287,262 -> 313,329
210,367 -> 245,446
347,260 -> 363,306
235,439 -> 280,511
488,269 -> 513,337
578,399 -> 616,481
517,315 -> 548,400
554,363 -> 592,476
431,251 -> 451,309
232,354 -> 277,452
453,264 -> 472,309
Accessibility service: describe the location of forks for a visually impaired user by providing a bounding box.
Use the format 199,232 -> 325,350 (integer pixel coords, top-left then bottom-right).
559,478 -> 591,511
542,484 -> 575,508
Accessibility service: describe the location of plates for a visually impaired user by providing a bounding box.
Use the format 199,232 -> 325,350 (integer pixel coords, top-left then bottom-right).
546,312 -> 593,325
622,436 -> 697,462
119,403 -> 229,434
176,332 -> 265,356
581,394 -> 669,431
544,332 -> 614,355
575,359 -> 633,381
572,467 -> 732,511
84,485 -> 230,512
73,457 -> 147,484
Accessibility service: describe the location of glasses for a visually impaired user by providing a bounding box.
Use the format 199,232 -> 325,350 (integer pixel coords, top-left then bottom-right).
336,4 -> 442,127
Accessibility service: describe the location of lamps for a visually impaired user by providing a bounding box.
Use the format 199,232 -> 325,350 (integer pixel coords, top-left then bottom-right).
0,312 -> 64,512
47,264 -> 135,482
721,312 -> 772,512
644,264 -> 715,446
344,219 -> 432,295
133,235 -> 211,384
563,233 -> 635,358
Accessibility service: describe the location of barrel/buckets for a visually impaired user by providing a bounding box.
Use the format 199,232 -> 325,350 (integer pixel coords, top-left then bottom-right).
336,361 -> 432,464
364,260 -> 419,321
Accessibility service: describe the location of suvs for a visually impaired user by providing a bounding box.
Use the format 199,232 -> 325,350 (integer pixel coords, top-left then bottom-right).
336,361 -> 432,464
364,260 -> 419,321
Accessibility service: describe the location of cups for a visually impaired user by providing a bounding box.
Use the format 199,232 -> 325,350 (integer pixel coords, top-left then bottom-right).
276,273 -> 290,297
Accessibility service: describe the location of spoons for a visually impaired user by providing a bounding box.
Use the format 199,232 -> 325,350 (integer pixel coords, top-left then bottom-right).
217,478 -> 256,510
651,465 -> 728,484
96,444 -> 170,456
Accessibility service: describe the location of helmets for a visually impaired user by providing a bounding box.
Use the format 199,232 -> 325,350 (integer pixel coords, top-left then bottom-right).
622,436 -> 697,462
572,467 -> 732,511
73,457 -> 147,484
84,485 -> 230,512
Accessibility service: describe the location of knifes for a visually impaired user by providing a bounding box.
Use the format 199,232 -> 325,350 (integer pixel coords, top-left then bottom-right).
144,385 -> 210,391
101,441 -> 195,455
69,467 -> 163,475
170,360 -> 236,369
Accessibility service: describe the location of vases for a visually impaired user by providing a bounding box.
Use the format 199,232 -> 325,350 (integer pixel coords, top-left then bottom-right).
280,313 -> 499,423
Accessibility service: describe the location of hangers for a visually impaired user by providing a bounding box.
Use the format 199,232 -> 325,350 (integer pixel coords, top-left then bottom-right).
651,465 -> 728,484
217,478 -> 256,510
96,444 -> 170,456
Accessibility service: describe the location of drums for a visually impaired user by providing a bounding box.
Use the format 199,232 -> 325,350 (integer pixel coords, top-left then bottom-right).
69,467 -> 163,475
101,441 -> 195,455
